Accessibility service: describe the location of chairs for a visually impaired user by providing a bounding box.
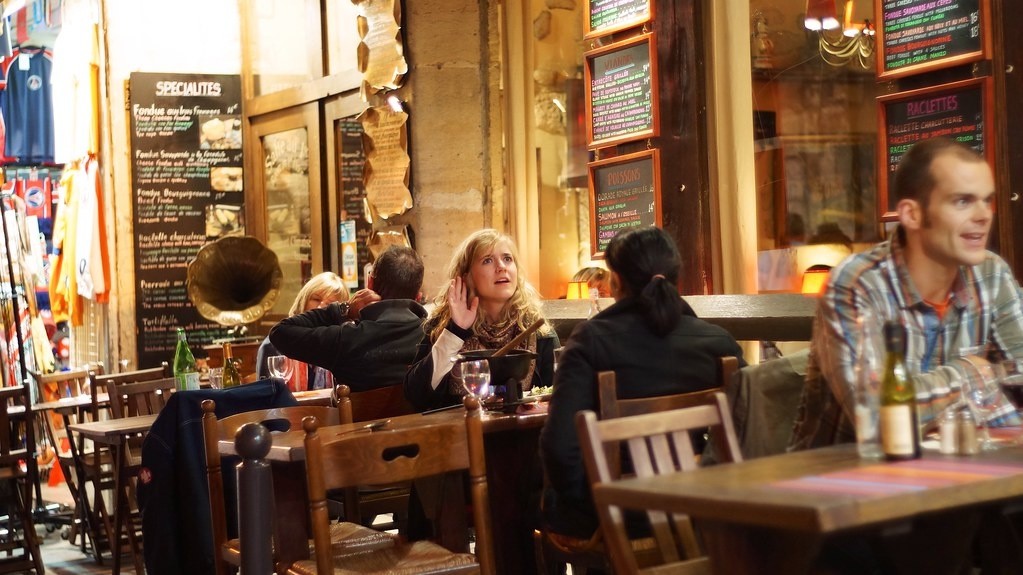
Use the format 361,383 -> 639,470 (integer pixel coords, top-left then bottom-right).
0,350 -> 810,575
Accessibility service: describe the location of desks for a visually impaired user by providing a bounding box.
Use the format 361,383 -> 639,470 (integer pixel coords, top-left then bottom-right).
219,398 -> 549,574
594,443 -> 1023,575
68,388 -> 332,575
8,393 -> 128,545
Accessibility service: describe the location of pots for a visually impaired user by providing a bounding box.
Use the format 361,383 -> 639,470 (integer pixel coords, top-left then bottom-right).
451,346 -> 538,384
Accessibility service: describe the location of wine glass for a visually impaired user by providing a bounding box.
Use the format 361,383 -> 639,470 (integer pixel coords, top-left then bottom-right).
962,364 -> 1003,452
460,359 -> 491,420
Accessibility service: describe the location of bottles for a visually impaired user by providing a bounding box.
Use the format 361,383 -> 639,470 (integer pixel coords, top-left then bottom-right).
957,411 -> 980,455
851,314 -> 884,458
939,410 -> 958,454
222,342 -> 239,389
173,328 -> 200,391
588,289 -> 601,320
879,320 -> 920,460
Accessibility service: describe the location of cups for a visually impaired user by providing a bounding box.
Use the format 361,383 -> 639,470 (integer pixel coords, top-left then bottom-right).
996,358 -> 1023,420
208,367 -> 222,391
266,354 -> 293,384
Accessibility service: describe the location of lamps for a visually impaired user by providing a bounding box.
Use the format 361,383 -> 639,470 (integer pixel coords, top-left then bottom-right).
803,0 -> 875,69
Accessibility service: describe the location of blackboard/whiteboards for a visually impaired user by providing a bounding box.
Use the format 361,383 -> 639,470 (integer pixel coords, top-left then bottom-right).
129,72 -> 248,370
338,117 -> 411,294
583,0 -> 655,40
874,0 -> 994,83
582,31 -> 659,151
875,76 -> 997,223
587,148 -> 663,261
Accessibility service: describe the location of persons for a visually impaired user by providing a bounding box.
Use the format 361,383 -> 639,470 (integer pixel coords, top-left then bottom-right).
789,214 -> 844,246
788,138 -> 1023,451
403,229 -> 561,541
269,247 -> 428,391
543,227 -> 747,575
256,272 -> 350,391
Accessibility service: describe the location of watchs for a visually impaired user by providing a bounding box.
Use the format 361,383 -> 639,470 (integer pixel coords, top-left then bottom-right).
339,302 -> 350,321
